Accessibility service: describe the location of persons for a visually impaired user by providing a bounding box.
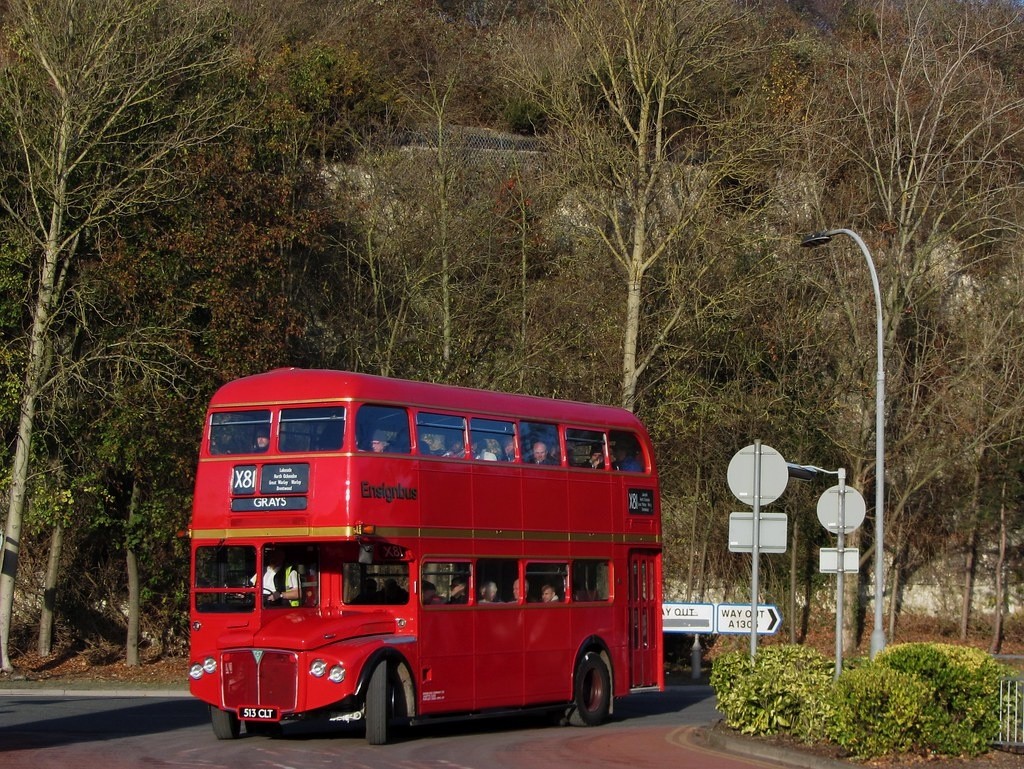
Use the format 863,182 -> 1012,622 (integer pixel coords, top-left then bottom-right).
249,545 -> 561,607
254,428 -> 643,474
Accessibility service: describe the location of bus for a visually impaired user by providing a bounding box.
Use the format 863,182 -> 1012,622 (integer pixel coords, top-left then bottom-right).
176,366 -> 666,748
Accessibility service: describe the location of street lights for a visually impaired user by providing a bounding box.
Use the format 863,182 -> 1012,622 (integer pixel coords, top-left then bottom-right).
798,226 -> 889,665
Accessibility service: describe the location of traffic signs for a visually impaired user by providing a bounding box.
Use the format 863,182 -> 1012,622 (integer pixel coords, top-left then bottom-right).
660,601 -> 783,636
